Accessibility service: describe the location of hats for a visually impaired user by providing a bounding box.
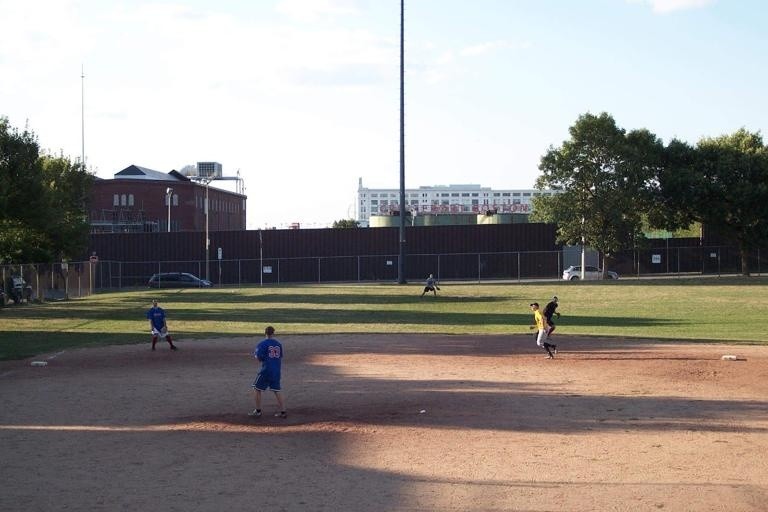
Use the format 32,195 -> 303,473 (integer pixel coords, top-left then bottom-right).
530,303 -> 539,307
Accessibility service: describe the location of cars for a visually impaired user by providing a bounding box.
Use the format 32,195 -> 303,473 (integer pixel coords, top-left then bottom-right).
562,265 -> 617,281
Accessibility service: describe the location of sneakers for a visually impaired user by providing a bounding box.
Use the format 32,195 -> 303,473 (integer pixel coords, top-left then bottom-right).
274,411 -> 287,417
151,348 -> 156,353
248,409 -> 262,416
171,345 -> 177,351
544,344 -> 560,359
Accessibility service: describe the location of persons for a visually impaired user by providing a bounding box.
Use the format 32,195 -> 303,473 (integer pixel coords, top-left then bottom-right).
246,325 -> 288,420
146,297 -> 179,353
527,302 -> 557,361
540,295 -> 561,338
419,273 -> 440,299
0,270 -> 33,308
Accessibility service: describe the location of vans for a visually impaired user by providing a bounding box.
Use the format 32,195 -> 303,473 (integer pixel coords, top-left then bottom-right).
148,272 -> 214,290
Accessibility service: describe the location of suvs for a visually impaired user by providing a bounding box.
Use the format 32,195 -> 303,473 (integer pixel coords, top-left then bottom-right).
5,277 -> 33,303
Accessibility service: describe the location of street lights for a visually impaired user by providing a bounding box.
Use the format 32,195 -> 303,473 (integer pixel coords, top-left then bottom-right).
203,178 -> 210,282
166,188 -> 174,231
